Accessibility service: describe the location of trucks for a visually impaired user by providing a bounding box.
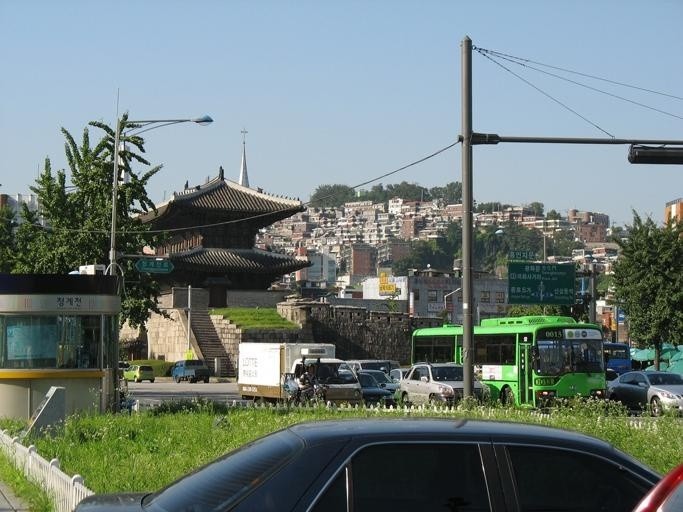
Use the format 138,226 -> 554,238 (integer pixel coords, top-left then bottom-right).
171,360 -> 211,383
238,340 -> 363,407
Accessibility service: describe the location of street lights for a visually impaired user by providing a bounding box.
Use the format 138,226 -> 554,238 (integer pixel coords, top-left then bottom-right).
109,115 -> 213,275
444,288 -> 462,308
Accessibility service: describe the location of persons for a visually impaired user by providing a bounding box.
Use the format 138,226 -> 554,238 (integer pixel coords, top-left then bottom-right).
296,363 -> 328,407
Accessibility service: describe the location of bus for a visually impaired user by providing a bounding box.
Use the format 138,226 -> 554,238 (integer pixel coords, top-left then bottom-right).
411,318 -> 610,413
537,341 -> 632,374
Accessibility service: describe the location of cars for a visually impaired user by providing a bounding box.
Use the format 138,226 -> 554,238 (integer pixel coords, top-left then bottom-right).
119,361 -> 154,382
398,361 -> 492,409
337,357 -> 410,409
605,370 -> 683,415
74,420 -> 683,512
